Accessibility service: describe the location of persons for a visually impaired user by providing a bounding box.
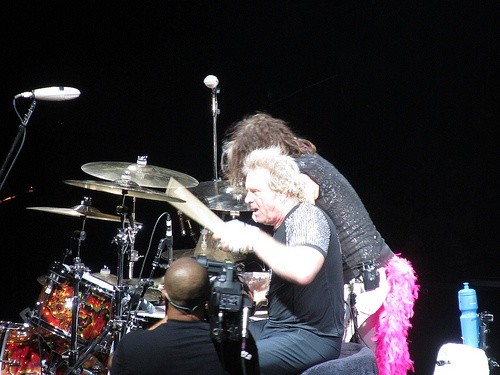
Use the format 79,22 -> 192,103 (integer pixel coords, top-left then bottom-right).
219,115 -> 421,375
108,256 -> 261,375
212,144 -> 345,375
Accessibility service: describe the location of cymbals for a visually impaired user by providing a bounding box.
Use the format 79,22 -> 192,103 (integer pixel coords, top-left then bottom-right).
25,202 -> 142,226
160,247 -> 248,264
80,159 -> 200,190
195,178 -> 259,214
62,179 -> 189,204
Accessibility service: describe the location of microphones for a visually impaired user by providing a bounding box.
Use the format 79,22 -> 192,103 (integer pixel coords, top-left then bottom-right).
166,214 -> 174,264
204,75 -> 219,89
179,210 -> 186,236
21,86 -> 81,101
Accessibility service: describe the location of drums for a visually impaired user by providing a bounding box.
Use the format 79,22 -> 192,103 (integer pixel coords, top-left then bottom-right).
0,320 -> 108,375
27,262 -> 115,344
129,305 -> 177,331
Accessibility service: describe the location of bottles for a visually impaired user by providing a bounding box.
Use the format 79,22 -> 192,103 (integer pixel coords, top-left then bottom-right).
457,282 -> 480,347
